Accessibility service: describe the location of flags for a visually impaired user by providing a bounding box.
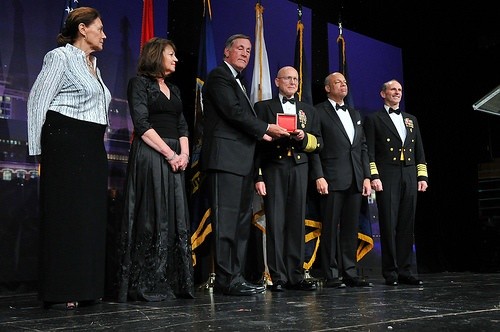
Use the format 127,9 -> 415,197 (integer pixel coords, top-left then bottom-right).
294,20 -> 305,101
203,0 -> 217,76
250,3 -> 273,106
337,34 -> 350,105
140,0 -> 155,54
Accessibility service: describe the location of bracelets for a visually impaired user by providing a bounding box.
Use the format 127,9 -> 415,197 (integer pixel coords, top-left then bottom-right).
182,153 -> 190,160
165,152 -> 176,160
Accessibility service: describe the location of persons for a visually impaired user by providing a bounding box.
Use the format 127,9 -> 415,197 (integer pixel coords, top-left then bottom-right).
254,66 -> 323,292
308,73 -> 373,289
111,38 -> 198,303
200,33 -> 291,296
27,7 -> 112,308
363,79 -> 429,286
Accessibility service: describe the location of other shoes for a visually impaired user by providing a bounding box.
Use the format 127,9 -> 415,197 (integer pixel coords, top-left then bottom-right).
50,301 -> 79,310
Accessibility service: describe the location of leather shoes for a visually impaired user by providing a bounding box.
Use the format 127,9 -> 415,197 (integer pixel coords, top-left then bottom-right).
385,279 -> 399,286
350,278 -> 374,288
326,279 -> 347,288
286,278 -> 317,291
272,280 -> 287,292
398,274 -> 424,285
224,280 -> 265,296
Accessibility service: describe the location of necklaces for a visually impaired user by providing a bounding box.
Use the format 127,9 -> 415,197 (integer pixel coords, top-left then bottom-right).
87,60 -> 93,68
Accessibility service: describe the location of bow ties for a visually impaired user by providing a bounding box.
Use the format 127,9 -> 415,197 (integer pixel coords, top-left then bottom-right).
389,108 -> 401,115
236,74 -> 245,80
336,103 -> 346,112
282,96 -> 296,104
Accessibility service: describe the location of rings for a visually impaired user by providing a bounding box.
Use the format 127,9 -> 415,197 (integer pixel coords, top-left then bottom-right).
183,167 -> 186,170
319,191 -> 322,193
